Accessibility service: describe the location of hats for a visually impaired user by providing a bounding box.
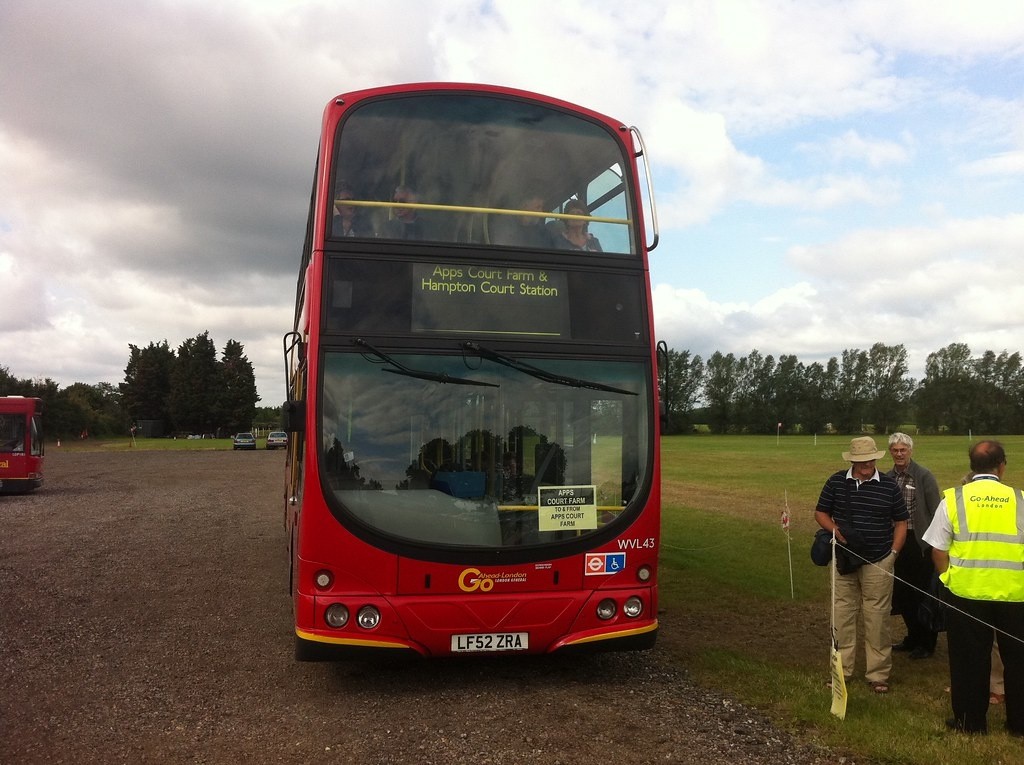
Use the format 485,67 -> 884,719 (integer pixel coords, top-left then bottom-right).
841,436 -> 887,463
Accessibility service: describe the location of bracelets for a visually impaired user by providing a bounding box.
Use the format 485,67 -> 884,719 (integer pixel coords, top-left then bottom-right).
891,548 -> 899,558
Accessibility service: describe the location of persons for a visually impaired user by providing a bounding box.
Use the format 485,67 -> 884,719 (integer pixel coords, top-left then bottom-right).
550,197 -> 604,251
945,470 -> 1006,704
494,191 -> 554,248
815,436 -> 911,692
376,184 -> 436,240
883,431 -> 944,659
920,439 -> 1024,736
495,451 -> 535,545
332,190 -> 376,238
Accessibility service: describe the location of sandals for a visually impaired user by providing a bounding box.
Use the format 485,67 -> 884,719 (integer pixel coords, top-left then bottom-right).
869,681 -> 890,693
824,675 -> 852,690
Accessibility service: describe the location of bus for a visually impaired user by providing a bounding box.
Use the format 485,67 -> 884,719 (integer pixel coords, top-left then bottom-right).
0,395 -> 46,493
281,80 -> 672,664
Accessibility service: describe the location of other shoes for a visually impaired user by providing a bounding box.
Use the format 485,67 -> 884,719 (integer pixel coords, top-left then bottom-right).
944,718 -> 982,734
908,646 -> 932,659
889,636 -> 915,651
1002,719 -> 1024,737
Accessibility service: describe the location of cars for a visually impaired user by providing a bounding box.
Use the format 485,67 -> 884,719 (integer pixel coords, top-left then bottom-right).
266,432 -> 289,450
234,433 -> 257,450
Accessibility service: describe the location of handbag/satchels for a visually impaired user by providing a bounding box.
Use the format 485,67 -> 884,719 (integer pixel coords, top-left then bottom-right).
835,550 -> 863,576
811,529 -> 837,567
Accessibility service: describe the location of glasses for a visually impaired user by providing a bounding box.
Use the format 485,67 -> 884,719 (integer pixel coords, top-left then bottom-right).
338,197 -> 357,201
393,199 -> 416,203
